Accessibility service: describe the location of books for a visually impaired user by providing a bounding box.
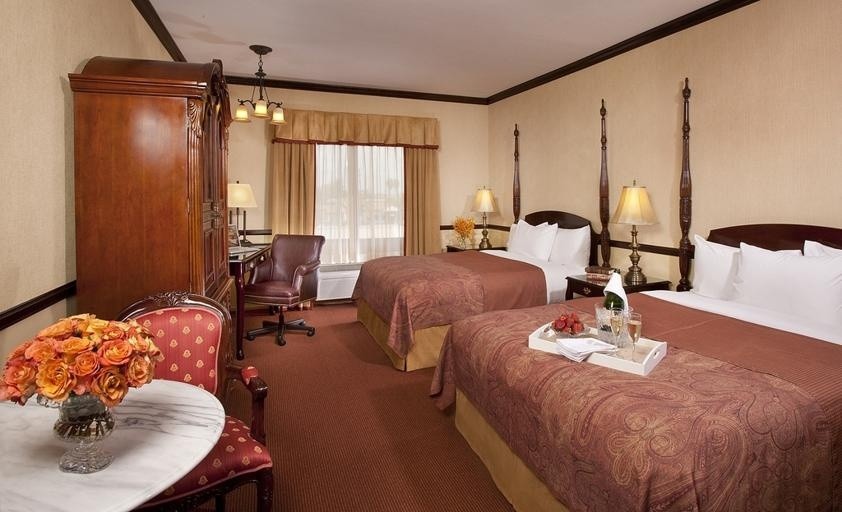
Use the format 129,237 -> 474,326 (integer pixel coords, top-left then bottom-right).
585,264 -> 621,282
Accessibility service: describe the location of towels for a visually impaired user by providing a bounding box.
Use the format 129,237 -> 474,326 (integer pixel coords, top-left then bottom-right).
604,273 -> 630,320
556,338 -> 618,362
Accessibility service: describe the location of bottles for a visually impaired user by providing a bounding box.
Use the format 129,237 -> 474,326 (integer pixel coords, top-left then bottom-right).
600,269 -> 623,332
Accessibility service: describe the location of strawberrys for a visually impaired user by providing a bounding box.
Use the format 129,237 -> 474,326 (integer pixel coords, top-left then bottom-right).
552,312 -> 583,333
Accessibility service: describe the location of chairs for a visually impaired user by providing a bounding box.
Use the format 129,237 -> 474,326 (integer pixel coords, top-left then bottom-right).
245,234 -> 325,346
108,291 -> 276,510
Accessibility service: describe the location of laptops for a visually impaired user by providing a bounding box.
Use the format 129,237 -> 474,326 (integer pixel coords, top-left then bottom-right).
228,224 -> 261,255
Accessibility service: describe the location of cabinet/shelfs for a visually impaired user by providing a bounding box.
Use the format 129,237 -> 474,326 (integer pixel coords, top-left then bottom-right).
67,55 -> 240,374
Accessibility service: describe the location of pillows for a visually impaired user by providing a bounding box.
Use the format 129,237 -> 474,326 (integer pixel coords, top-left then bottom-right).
516,221 -> 558,263
797,240 -> 842,342
735,240 -> 801,318
688,235 -> 746,305
546,226 -> 590,267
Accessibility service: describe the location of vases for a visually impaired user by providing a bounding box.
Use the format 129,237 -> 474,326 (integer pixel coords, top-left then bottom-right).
50,402 -> 115,474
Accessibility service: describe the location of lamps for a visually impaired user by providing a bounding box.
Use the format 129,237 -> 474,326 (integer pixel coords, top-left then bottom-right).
611,181 -> 656,283
234,45 -> 290,126
220,178 -> 257,247
472,186 -> 500,252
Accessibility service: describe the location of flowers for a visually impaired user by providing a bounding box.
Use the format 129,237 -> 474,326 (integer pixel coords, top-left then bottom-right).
3,314 -> 160,400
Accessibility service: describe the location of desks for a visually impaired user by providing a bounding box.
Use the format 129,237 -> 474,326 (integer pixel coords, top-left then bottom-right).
0,368 -> 227,512
228,242 -> 272,361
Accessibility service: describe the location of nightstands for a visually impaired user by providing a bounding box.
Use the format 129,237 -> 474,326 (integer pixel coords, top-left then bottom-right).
446,242 -> 507,253
565,273 -> 674,308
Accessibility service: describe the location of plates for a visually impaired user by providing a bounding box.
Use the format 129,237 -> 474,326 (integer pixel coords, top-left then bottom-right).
547,326 -> 592,339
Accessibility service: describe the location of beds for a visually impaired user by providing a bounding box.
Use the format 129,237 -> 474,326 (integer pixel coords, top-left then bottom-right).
430,224 -> 842,512
351,211 -> 612,372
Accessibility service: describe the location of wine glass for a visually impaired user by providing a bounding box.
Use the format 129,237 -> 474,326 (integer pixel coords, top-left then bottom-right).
609,308 -> 625,355
626,312 -> 642,361
